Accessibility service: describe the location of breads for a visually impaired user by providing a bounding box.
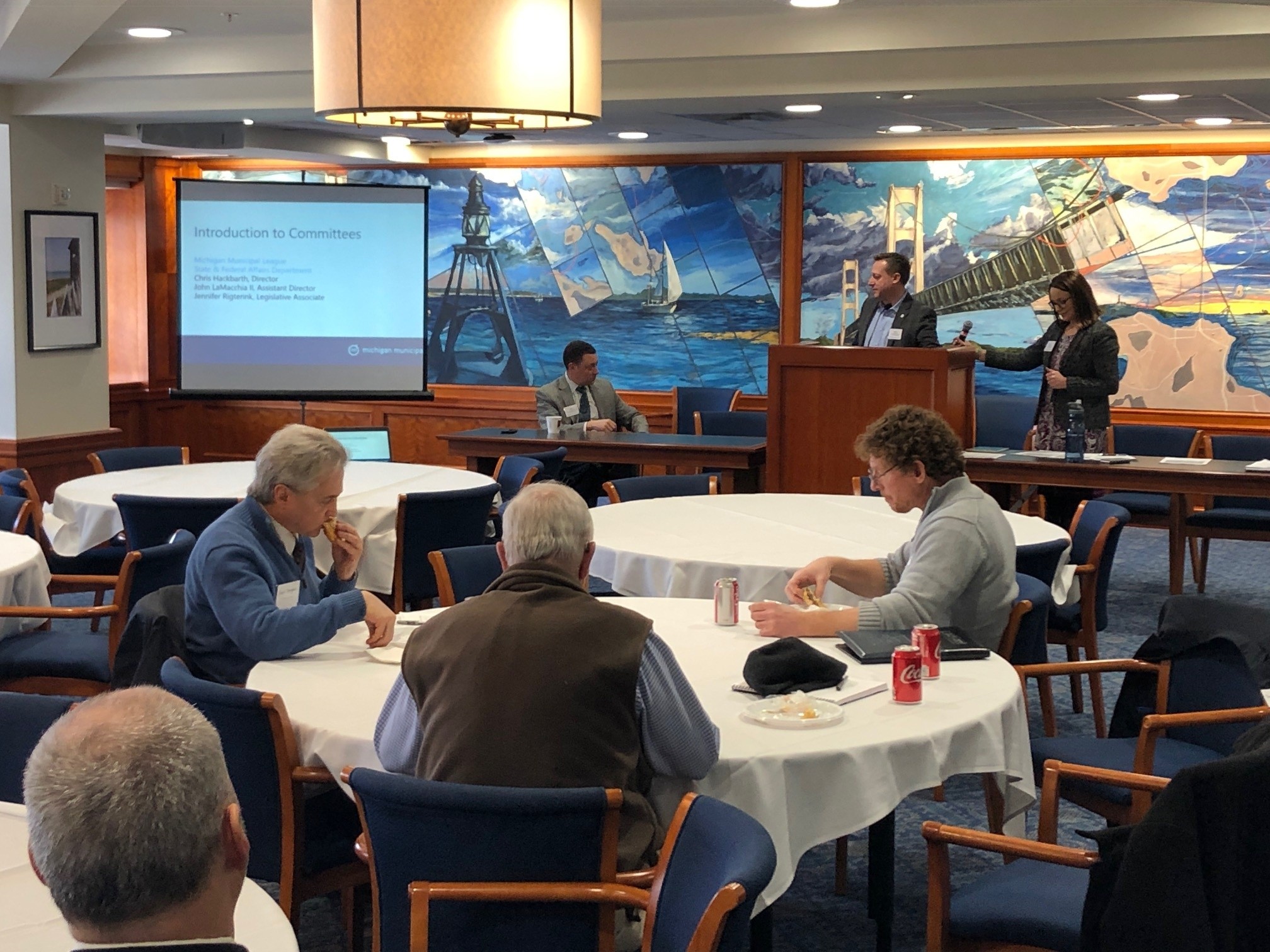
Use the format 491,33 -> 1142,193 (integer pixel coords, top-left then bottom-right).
322,516 -> 340,544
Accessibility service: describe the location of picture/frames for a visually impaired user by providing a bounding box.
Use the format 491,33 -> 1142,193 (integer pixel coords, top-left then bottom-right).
24,210 -> 102,352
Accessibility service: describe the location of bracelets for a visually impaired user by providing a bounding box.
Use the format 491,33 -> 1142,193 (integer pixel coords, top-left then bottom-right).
975,346 -> 981,359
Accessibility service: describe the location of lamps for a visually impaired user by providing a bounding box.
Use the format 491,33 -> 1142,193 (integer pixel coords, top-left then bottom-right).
311,0 -> 601,140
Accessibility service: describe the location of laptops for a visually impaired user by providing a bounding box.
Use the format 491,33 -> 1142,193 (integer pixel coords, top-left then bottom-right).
324,427 -> 392,462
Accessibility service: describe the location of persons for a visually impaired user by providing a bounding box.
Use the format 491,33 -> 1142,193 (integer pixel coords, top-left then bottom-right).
183,424 -> 396,873
535,340 -> 649,508
748,406 -> 1020,651
835,253 -> 940,347
957,271 -> 1120,532
374,480 -> 720,935
22,686 -> 250,952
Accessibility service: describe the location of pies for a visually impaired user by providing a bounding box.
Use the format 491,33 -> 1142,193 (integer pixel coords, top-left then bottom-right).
803,588 -> 823,608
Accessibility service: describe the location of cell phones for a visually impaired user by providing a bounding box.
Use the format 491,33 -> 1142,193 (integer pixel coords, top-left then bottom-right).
501,429 -> 517,434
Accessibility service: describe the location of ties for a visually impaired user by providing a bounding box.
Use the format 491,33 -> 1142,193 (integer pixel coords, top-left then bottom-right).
575,385 -> 594,425
289,533 -> 309,573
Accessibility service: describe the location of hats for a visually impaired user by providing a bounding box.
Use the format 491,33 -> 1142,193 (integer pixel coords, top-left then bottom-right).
741,636 -> 849,698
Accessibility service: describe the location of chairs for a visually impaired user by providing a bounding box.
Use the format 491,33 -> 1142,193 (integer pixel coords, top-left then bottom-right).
408,790 -> 777,952
921,758 -> 1174,952
602,386 -> 768,505
852,475 -> 882,498
0,690 -> 91,805
492,454 -> 544,503
161,656 -> 363,952
1013,596 -> 1270,850
341,763 -> 622,952
0,495 -> 34,534
1185,434 -> 1270,595
393,483 -> 501,617
111,495 -> 245,556
512,446 -> 567,483
135,584 -> 185,686
0,528 -> 196,693
1026,499 -> 1131,713
0,468 -> 118,633
429,545 -> 505,606
1094,425 -> 1201,595
87,446 -> 189,473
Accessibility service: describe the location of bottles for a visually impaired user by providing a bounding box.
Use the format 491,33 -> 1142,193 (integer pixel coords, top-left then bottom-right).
1064,402 -> 1084,464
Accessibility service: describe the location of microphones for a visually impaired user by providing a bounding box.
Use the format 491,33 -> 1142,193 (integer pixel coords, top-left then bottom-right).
959,320 -> 973,341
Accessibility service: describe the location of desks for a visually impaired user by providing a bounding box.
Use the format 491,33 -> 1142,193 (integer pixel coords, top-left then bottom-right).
1108,404 -> 1270,458
0,800 -> 300,952
587,493 -> 1072,608
54,461 -> 497,544
963,447 -> 1270,595
248,596 -> 1018,952
435,426 -> 770,496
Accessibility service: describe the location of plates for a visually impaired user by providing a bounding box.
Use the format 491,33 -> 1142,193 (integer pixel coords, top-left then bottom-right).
743,697 -> 845,728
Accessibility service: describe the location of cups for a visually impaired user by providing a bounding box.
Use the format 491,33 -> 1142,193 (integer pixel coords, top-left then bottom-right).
546,416 -> 563,433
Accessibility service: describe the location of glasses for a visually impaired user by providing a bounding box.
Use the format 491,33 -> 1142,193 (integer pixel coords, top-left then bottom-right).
866,457 -> 915,484
1047,295 -> 1075,309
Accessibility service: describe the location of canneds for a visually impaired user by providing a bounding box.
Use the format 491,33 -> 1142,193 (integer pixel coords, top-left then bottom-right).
911,624 -> 941,680
714,578 -> 740,626
891,645 -> 923,705
